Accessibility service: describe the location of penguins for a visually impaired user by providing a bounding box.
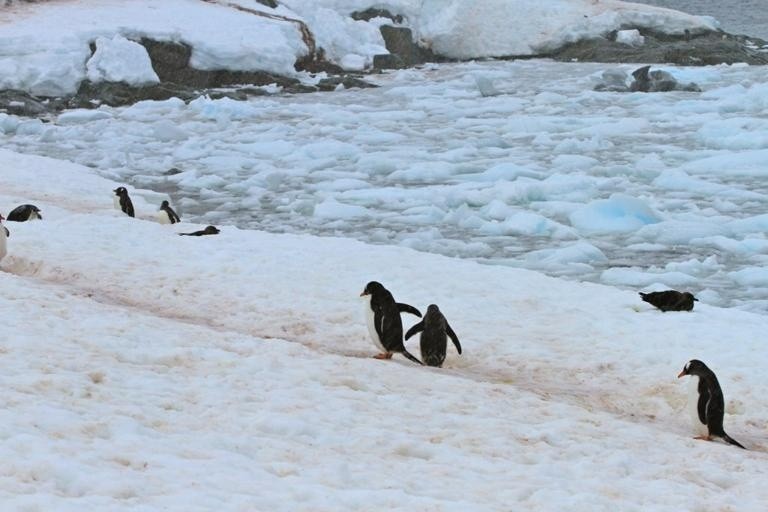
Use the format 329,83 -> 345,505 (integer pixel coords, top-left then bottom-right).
405,304 -> 462,368
178,226 -> 220,236
0,214 -> 9,261
7,205 -> 42,221
677,359 -> 745,449
113,187 -> 134,218
157,200 -> 180,225
360,281 -> 424,365
638,290 -> 699,313
